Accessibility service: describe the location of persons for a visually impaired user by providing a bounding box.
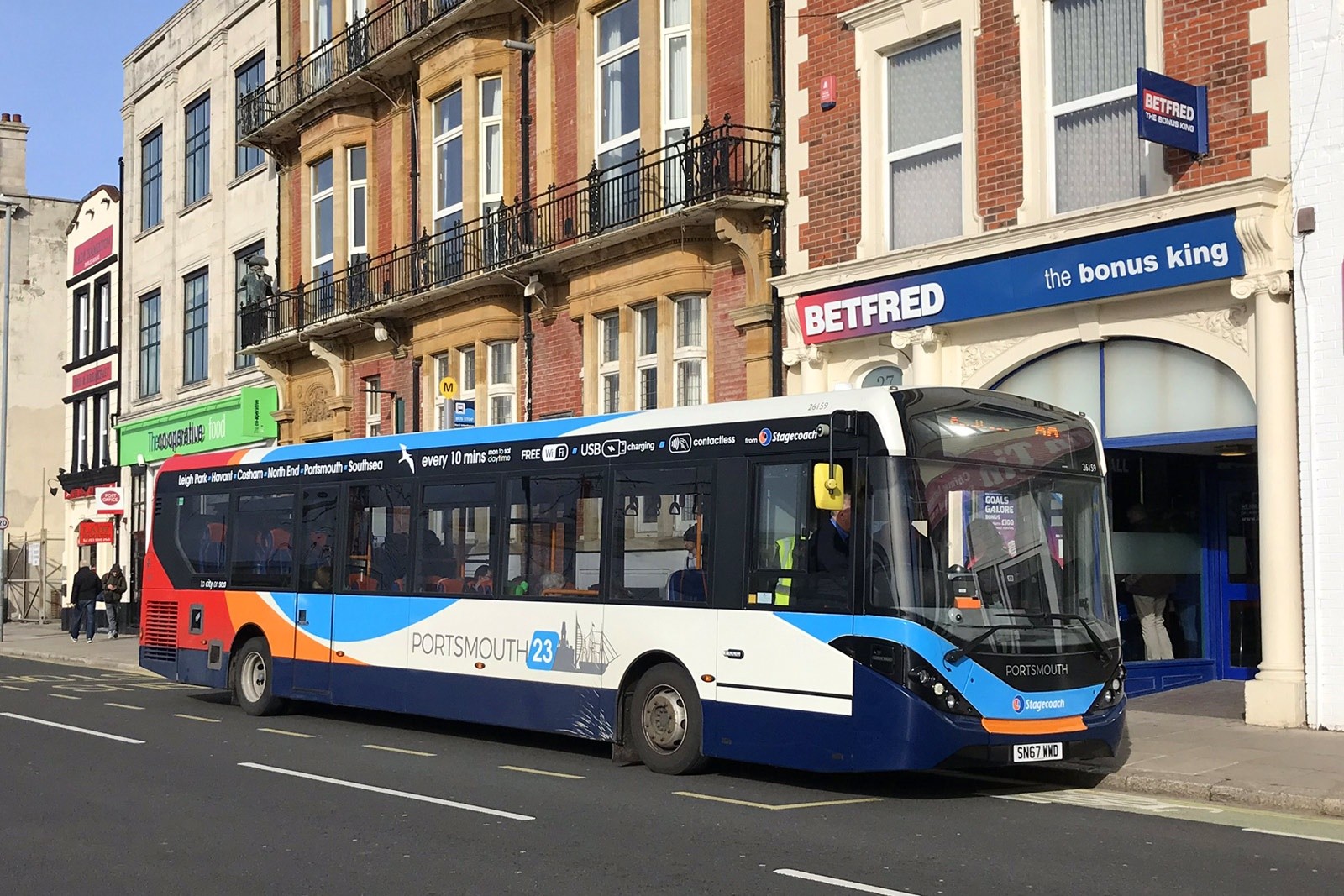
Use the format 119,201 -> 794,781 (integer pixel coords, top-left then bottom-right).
964,519 -> 1017,603
807,492 -> 935,615
1120,503 -> 1202,662
682,524 -> 702,569
306,540 -> 565,598
69,560 -> 102,643
102,564 -> 127,639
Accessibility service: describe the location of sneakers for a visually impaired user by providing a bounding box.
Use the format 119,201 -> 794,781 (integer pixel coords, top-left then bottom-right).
70,635 -> 78,643
86,638 -> 92,644
108,631 -> 113,639
112,629 -> 119,638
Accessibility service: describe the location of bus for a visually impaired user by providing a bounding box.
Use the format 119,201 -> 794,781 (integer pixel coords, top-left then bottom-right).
139,385 -> 1127,772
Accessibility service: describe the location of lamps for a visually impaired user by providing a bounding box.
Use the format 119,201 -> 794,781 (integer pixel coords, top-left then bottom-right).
79,464 -> 89,471
101,198 -> 112,205
48,478 -> 60,496
72,220 -> 79,228
86,210 -> 94,216
59,468 -> 66,475
374,322 -> 390,342
524,268 -> 546,298
102,459 -> 111,467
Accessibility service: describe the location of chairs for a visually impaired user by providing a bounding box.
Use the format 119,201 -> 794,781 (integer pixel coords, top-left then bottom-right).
769,532 -> 806,605
190,518 -> 494,594
663,568 -> 708,602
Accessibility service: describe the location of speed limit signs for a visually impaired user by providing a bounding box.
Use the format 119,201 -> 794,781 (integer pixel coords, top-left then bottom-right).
0,517 -> 9,530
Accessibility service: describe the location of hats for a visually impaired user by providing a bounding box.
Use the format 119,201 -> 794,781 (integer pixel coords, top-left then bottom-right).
683,526 -> 696,542
111,564 -> 122,572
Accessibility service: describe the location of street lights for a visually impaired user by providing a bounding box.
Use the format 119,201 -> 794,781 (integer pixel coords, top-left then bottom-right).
0,199 -> 32,642
